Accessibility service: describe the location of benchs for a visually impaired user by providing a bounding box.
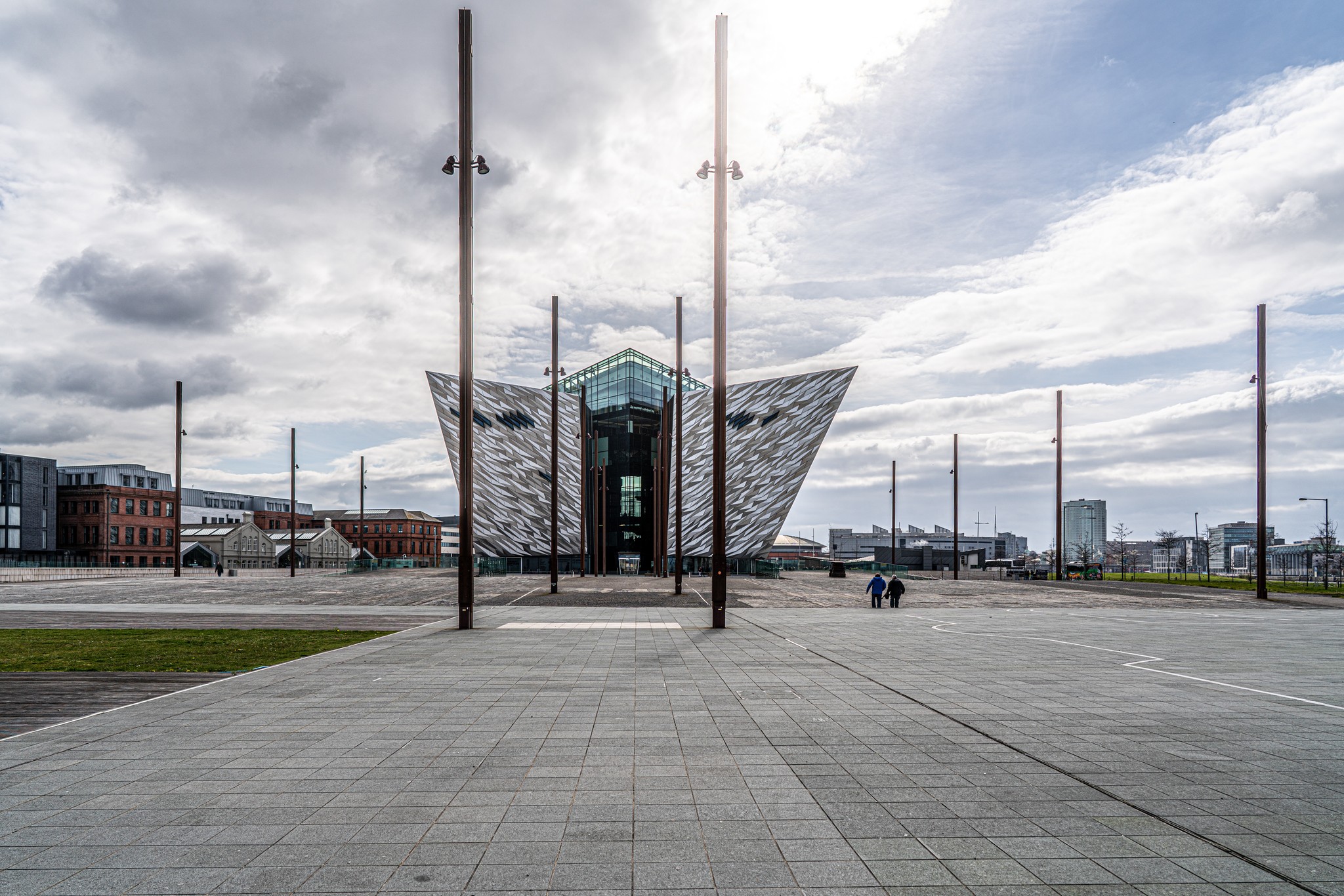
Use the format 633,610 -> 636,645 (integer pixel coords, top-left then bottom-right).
750,573 -> 756,577
660,573 -> 675,577
708,573 -> 730,578
645,573 -> 654,577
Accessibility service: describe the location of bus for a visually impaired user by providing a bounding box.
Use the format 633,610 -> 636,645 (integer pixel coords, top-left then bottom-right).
985,559 -> 1026,577
1061,561 -> 1103,581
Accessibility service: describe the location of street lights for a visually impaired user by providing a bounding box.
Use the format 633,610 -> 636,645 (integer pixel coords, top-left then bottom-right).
1063,505 -> 1087,581
1079,505 -> 1096,562
975,512 -> 989,549
1299,498 -> 1328,590
1195,512 -> 1201,581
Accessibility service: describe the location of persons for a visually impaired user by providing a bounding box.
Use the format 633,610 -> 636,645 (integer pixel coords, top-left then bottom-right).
1024,569 -> 1028,580
865,573 -> 886,608
1026,570 -> 1030,580
886,574 -> 905,608
1029,570 -> 1034,580
783,565 -> 832,571
701,565 -> 705,577
629,568 -> 631,571
215,562 -> 224,576
698,567 -> 702,577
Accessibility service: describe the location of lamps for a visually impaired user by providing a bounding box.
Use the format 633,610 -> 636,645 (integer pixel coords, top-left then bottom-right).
181,562 -> 183,565
358,534 -> 360,536
425,535 -> 427,537
277,563 -> 279,565
213,563 -> 215,565
302,563 -> 304,565
378,535 -> 382,536
347,535 -> 351,536
391,534 -> 394,536
162,562 -> 165,565
122,562 -> 125,565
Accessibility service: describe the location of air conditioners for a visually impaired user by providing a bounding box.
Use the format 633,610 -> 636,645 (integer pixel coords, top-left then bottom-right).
961,555 -> 967,564
